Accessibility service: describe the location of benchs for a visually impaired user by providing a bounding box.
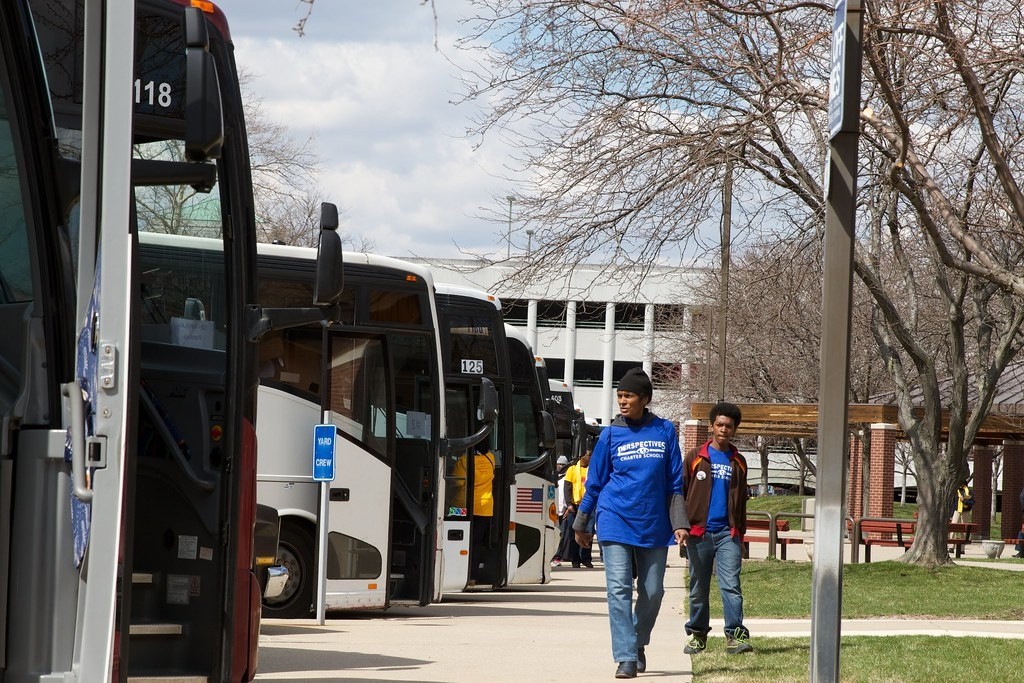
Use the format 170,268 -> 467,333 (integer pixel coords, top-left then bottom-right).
1003,525 -> 1024,544
741,512 -> 978,564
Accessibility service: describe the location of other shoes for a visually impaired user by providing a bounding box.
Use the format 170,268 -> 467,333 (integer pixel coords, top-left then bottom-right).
572,561 -> 581,567
551,560 -> 561,567
581,560 -> 594,568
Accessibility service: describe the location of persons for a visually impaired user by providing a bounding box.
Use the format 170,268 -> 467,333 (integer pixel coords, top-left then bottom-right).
682,402 -> 753,654
572,367 -> 691,678
551,449 -> 595,569
449,423 -> 496,585
946,480 -> 976,553
1012,488 -> 1024,558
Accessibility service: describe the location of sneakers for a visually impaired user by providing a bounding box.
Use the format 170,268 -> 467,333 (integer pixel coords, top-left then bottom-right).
684,633 -> 707,654
727,627 -> 752,654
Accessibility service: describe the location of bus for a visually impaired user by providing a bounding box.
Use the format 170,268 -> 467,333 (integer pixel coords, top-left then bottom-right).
0,0 -> 345,683
135,231 -> 498,621
433,283 -> 606,604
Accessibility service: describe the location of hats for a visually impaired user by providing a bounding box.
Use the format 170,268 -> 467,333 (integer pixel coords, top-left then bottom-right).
557,456 -> 568,464
617,367 -> 652,399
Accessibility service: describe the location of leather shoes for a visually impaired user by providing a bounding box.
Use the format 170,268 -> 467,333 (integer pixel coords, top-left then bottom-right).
615,660 -> 638,678
636,647 -> 646,672
